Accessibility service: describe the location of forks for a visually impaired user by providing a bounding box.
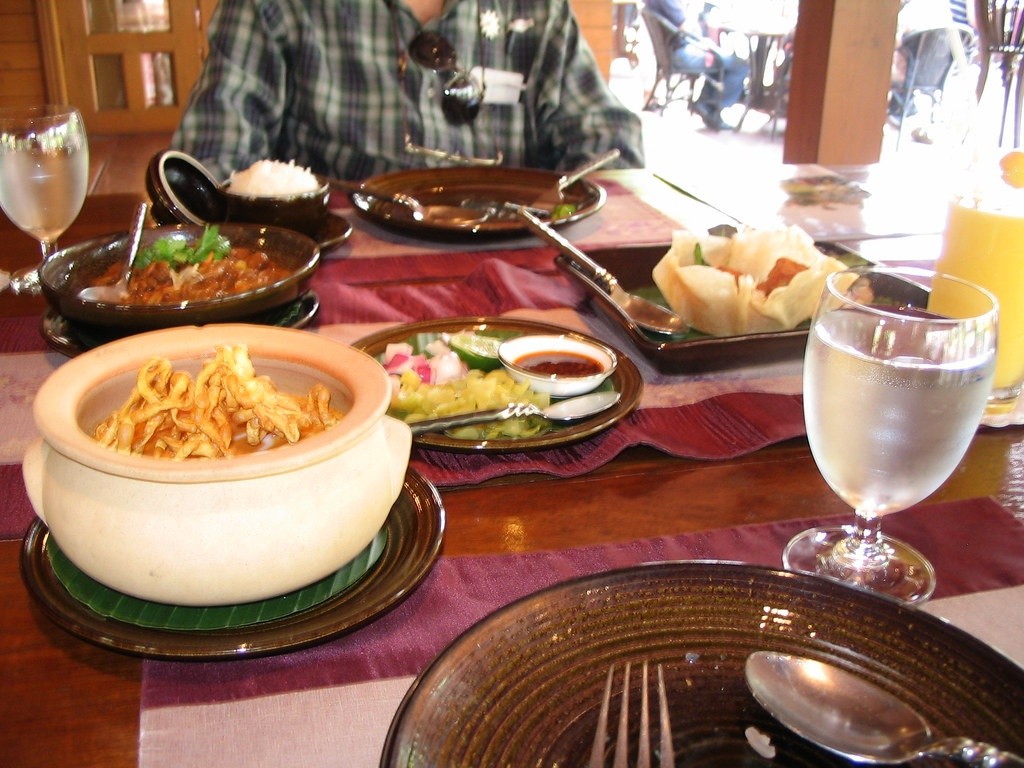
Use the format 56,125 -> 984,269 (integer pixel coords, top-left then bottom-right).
588,659 -> 675,768
511,148 -> 620,220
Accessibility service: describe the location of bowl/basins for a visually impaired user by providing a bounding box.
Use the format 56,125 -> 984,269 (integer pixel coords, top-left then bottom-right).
218,174 -> 329,241
20,322 -> 413,606
377,560 -> 1024,768
498,333 -> 618,399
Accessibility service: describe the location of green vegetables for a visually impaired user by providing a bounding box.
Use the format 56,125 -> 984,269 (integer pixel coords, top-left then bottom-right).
131,222 -> 231,269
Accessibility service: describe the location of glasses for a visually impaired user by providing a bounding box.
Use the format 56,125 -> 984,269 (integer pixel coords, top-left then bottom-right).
400,52 -> 505,166
409,30 -> 483,126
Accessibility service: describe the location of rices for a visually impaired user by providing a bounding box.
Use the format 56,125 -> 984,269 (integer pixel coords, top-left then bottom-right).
225,158 -> 320,195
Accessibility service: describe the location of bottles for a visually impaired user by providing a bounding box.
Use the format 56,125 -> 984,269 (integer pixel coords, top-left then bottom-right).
933,152 -> 1024,428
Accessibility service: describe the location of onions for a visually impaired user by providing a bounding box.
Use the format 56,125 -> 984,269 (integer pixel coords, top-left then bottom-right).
380,341 -> 468,386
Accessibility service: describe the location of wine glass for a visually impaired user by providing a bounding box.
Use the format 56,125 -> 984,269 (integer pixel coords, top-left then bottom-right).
0,103 -> 90,295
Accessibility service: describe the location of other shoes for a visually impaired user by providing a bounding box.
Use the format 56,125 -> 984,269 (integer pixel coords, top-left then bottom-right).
691,101 -> 733,130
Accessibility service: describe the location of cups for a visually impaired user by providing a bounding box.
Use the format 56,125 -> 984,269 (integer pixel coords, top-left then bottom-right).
781,266 -> 1000,607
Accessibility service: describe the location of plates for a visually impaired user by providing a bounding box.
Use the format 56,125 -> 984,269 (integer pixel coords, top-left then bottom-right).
20,463 -> 445,657
350,316 -> 642,450
40,289 -> 320,358
318,213 -> 353,255
350,165 -> 606,231
554,236 -> 932,374
41,224 -> 325,320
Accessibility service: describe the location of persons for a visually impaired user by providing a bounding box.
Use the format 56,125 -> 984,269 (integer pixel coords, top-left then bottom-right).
888,0 -> 954,118
644,0 -> 751,131
171,1 -> 646,186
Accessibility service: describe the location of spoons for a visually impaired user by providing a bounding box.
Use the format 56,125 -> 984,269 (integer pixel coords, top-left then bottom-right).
514,203 -> 691,337
79,201 -> 148,304
312,175 -> 489,223
407,390 -> 620,435
746,651 -> 1024,768
